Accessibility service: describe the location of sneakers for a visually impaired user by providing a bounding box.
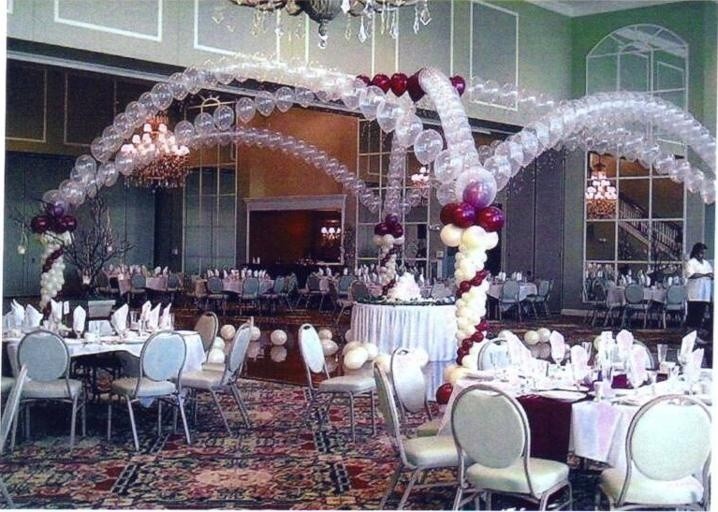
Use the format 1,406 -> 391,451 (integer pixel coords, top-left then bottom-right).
696,337 -> 708,345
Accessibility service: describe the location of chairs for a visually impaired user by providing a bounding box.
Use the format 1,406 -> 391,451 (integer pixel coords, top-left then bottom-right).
98,260 -> 376,324
595,393 -> 712,511
4,302 -> 253,451
374,363 -> 479,511
451,384 -> 573,511
295,324 -> 375,443
487,266 -> 689,328
386,329 -> 656,482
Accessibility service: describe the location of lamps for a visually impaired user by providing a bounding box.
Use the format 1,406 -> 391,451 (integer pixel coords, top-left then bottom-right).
316,216 -> 341,249
409,164 -> 431,199
585,149 -> 616,219
118,101 -> 193,192
211,0 -> 432,50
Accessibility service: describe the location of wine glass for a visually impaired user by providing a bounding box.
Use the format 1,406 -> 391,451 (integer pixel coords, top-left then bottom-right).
3,310 -> 176,343
496,340 -> 701,402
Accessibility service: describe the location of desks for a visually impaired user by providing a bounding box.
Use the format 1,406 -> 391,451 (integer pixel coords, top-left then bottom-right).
348,299 -> 457,362
444,367 -> 712,498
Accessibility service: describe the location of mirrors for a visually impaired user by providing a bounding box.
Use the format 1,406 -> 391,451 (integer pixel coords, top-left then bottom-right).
242,194 -> 347,266
352,113 -> 435,286
578,22 -> 692,311
180,87 -> 241,276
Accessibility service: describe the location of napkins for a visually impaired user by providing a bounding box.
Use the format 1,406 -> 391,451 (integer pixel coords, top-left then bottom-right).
680,330 -> 704,383
